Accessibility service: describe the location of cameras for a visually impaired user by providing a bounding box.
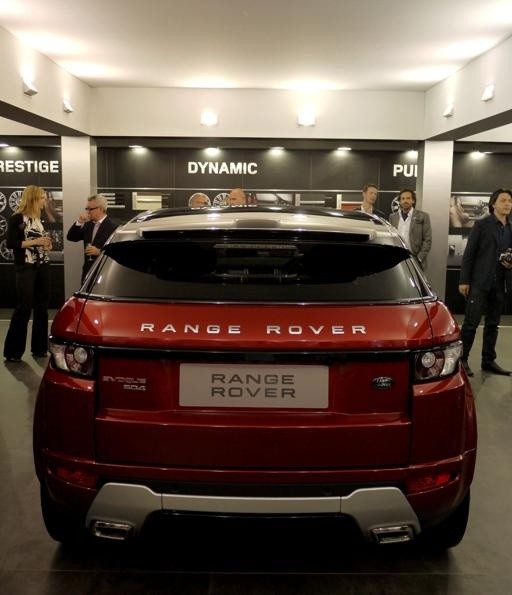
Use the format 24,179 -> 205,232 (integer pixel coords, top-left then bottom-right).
497,248 -> 512,262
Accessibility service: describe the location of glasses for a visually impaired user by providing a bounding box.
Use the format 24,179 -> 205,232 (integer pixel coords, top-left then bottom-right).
83,206 -> 99,211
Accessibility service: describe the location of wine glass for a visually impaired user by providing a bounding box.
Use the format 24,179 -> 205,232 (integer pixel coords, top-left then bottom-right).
88,244 -> 94,261
42,232 -> 50,251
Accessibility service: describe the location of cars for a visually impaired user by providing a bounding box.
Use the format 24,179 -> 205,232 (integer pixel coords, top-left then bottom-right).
32,203 -> 477,547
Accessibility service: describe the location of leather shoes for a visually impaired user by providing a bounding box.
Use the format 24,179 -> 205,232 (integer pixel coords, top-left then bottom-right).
464,360 -> 476,374
482,358 -> 511,377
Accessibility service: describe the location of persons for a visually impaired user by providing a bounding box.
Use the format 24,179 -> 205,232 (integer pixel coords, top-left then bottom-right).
457,188 -> 512,377
351,184 -> 385,218
2,185 -> 54,363
188,192 -> 211,209
225,186 -> 247,207
66,193 -> 116,286
389,189 -> 433,272
